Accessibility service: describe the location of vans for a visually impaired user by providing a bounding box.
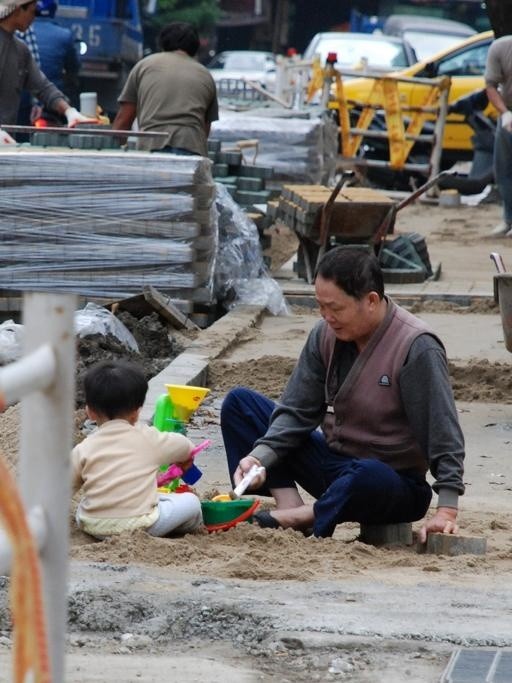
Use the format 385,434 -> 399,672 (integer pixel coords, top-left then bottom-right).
306,14 -> 488,70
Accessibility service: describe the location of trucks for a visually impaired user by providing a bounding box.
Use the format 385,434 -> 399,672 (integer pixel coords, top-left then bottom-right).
27,1 -> 143,83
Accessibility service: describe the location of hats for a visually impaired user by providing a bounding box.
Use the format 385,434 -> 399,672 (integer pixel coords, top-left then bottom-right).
0,0 -> 35,19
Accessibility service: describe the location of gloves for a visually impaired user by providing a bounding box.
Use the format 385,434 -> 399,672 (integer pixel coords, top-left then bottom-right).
500,109 -> 512,134
64,106 -> 99,129
0,129 -> 16,145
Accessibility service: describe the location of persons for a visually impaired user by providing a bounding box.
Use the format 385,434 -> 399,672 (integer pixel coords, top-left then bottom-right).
71,358 -> 200,538
219,244 -> 467,542
484,34 -> 510,236
29,3 -> 81,123
14,25 -> 42,124
110,20 -> 218,160
1,0 -> 99,146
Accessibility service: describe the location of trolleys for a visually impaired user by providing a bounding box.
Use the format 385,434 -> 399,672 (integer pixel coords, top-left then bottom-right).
283,174 -> 447,274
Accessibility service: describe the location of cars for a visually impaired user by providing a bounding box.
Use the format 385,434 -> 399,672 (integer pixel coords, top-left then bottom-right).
321,29 -> 504,158
203,47 -> 281,96
